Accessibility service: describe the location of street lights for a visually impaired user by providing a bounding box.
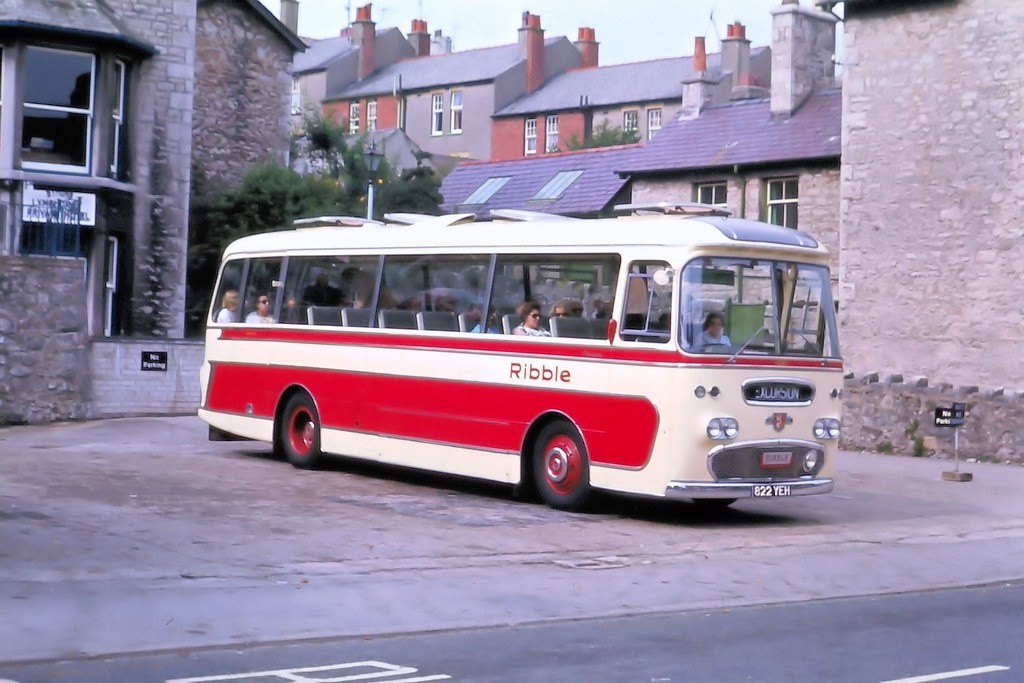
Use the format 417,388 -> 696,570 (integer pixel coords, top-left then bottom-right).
360,140 -> 384,220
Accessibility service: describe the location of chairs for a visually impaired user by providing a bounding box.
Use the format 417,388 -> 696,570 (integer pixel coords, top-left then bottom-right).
288,307 -> 645,341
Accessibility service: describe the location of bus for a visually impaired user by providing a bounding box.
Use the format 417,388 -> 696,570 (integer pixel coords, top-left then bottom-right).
198,202 -> 845,509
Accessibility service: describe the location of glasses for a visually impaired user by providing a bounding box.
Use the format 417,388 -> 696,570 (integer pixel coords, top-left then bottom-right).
529,313 -> 542,318
555,312 -> 566,317
260,301 -> 271,304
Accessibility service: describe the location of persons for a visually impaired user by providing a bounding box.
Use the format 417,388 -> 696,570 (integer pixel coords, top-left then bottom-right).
302,272 -> 342,307
245,294 -> 273,324
468,280 -> 503,334
217,291 -> 239,323
511,303 -> 550,337
552,298 -> 614,317
340,268 -> 459,311
695,312 -> 731,346
